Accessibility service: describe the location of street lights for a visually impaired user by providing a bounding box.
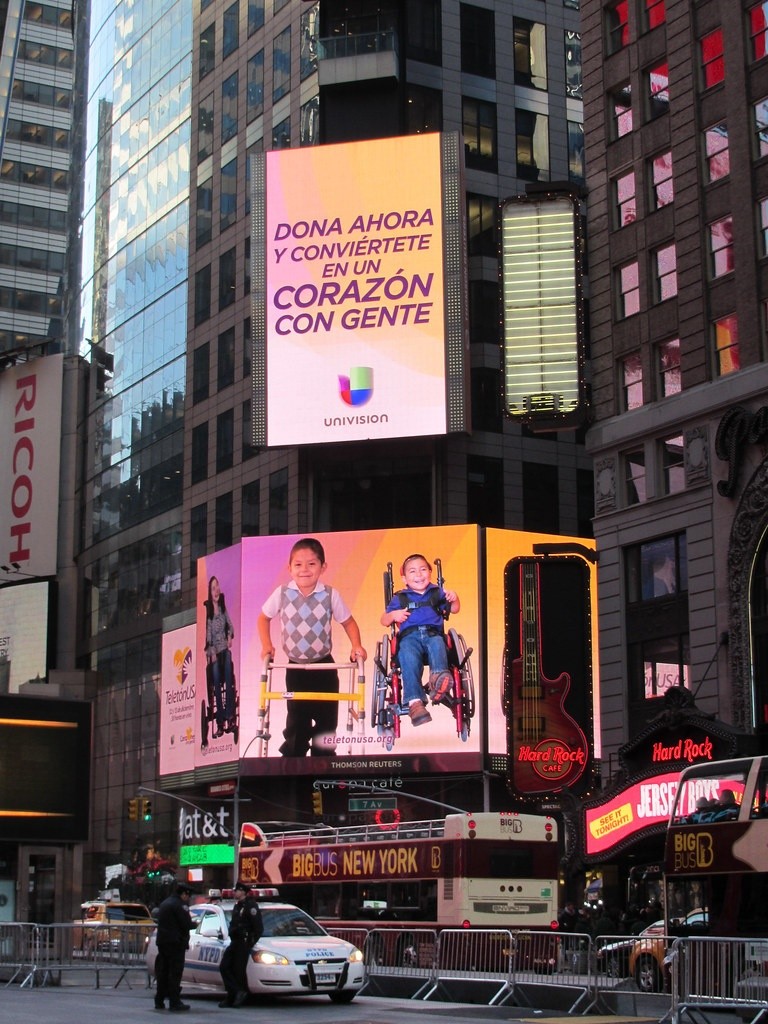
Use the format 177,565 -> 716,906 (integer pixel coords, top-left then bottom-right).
233,733 -> 273,888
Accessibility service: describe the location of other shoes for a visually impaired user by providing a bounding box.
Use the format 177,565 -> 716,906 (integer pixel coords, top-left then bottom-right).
155,1003 -> 165,1009
169,1002 -> 190,1011
233,991 -> 249,1007
219,993 -> 235,1007
215,720 -> 225,737
227,716 -> 236,731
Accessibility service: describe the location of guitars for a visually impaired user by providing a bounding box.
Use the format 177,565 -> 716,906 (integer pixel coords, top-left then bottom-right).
511,562 -> 588,795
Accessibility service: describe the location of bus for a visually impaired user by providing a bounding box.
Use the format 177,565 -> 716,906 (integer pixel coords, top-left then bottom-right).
237,811 -> 564,1002
628,756 -> 767,1018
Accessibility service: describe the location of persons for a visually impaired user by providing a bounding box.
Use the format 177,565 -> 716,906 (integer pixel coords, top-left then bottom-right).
155,882 -> 201,1010
379,554 -> 462,729
558,899 -> 662,977
258,539 -> 366,758
218,882 -> 265,1008
206,577 -> 235,736
687,789 -> 745,823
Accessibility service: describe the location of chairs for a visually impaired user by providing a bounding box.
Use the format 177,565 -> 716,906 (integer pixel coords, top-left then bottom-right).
262,913 -> 277,930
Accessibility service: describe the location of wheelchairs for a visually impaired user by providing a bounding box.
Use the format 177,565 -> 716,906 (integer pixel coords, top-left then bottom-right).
201,649 -> 239,746
370,558 -> 476,751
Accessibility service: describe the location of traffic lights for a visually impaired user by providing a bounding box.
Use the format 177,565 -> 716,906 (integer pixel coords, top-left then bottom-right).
311,791 -> 322,816
142,799 -> 151,822
128,798 -> 138,823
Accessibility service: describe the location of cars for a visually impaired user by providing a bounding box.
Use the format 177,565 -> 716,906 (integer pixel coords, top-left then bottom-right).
596,906 -> 710,994
72,888 -> 154,954
146,888 -> 366,1008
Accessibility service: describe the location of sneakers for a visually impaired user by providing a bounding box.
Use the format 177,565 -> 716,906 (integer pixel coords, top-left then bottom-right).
429,670 -> 453,704
409,700 -> 432,727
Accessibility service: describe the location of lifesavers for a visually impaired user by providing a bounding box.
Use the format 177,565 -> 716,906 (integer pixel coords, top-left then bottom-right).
375,809 -> 400,829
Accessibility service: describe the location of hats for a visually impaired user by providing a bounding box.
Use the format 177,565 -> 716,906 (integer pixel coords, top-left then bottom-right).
175,881 -> 192,893
234,881 -> 250,892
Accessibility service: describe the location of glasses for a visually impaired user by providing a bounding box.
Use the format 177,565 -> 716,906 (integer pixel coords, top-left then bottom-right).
185,890 -> 192,897
